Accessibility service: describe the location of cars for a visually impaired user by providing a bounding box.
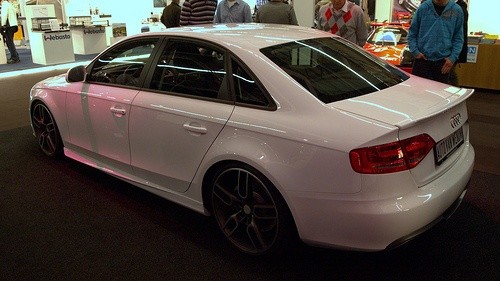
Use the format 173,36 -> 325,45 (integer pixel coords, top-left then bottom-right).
362,25 -> 413,68
28,21 -> 477,260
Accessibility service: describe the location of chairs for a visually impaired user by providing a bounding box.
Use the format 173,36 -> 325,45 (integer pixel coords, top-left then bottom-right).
168,46 -> 220,100
313,47 -> 350,78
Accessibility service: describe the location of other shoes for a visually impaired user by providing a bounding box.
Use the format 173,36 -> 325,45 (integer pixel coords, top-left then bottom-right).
8,57 -> 19,63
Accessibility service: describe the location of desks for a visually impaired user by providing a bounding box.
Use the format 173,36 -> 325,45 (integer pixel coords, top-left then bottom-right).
456,44 -> 500,90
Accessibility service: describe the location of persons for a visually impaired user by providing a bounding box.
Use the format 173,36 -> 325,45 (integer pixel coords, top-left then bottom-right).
314,0 -> 469,88
0,0 -> 20,64
160,0 -> 181,28
256,0 -> 299,66
212,0 -> 252,60
179,0 -> 218,55
